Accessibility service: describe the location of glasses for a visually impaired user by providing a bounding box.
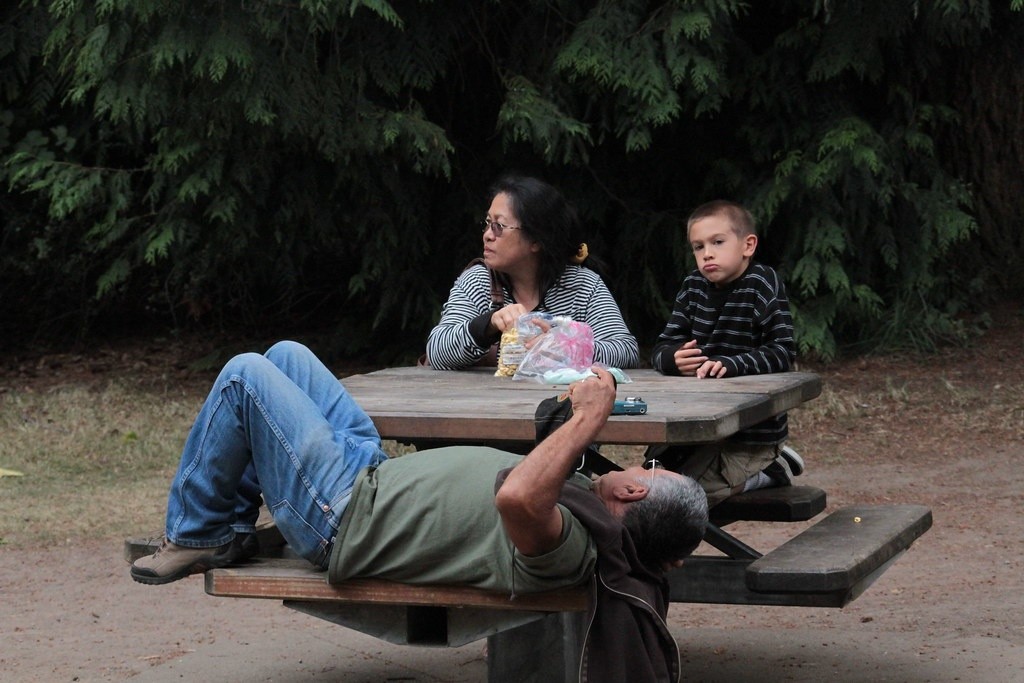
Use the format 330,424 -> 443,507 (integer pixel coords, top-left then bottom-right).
646,459 -> 662,487
480,219 -> 527,236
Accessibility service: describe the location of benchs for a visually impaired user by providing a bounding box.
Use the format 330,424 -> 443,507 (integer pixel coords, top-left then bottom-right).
747,501 -> 932,592
204,551 -> 592,611
733,485 -> 827,522
124,502 -> 287,566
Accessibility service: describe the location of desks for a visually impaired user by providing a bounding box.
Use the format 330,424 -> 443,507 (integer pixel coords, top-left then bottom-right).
336,359 -> 820,440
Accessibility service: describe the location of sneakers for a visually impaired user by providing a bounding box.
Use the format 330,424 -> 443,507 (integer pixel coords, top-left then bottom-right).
761,445 -> 805,485
130,535 -> 261,584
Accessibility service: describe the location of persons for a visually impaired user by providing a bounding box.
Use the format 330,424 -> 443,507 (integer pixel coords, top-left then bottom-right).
126,340 -> 707,594
425,175 -> 640,369
640,201 -> 806,499
